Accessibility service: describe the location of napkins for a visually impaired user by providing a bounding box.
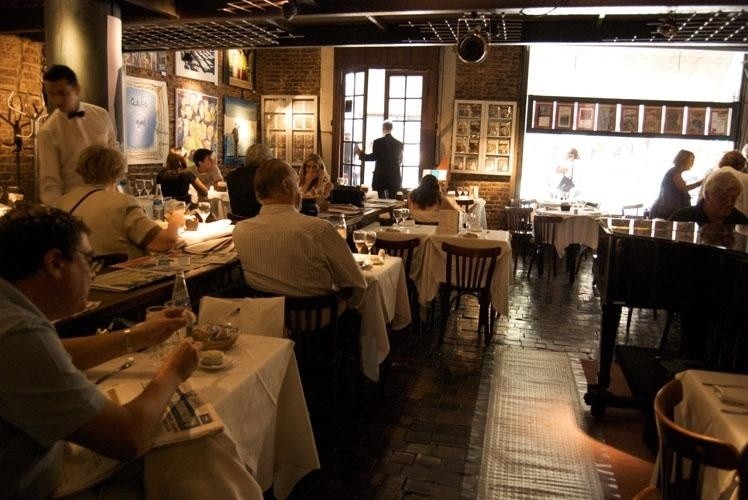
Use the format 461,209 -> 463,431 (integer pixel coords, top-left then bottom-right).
713,385 -> 746,405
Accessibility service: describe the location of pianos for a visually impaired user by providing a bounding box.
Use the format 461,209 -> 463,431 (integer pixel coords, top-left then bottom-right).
584,217 -> 748,417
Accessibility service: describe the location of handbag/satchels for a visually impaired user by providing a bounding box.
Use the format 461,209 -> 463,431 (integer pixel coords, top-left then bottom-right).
649,199 -> 665,219
325,189 -> 364,208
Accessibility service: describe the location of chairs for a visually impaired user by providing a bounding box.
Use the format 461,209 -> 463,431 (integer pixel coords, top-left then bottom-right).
500,186 -> 747,380
637,380 -> 739,495
0,186 -> 500,362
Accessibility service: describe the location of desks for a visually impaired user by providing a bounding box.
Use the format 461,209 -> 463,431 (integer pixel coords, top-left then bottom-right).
675,370 -> 747,499
62,333 -> 320,498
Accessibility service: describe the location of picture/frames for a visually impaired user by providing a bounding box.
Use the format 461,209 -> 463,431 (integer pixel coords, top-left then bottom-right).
126,76 -> 169,168
174,87 -> 219,170
223,49 -> 256,92
175,50 -> 219,87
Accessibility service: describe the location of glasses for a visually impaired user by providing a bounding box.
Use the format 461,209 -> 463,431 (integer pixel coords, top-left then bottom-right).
71,248 -> 104,274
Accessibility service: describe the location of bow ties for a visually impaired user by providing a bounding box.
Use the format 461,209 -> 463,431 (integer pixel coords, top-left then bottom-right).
68,111 -> 84,119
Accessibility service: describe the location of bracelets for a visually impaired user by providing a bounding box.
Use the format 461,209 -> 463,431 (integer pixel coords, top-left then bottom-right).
124,328 -> 133,354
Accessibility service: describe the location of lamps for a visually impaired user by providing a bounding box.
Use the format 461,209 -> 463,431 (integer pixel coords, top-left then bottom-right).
283,0 -> 305,21
656,12 -> 679,36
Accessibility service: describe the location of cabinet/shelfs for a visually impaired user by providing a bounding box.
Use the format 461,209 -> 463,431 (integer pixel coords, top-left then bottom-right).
451,99 -> 517,178
261,94 -> 318,167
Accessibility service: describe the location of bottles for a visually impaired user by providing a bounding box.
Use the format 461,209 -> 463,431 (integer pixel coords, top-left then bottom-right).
151,182 -> 165,223
170,269 -> 191,311
642,209 -> 649,219
334,212 -> 347,241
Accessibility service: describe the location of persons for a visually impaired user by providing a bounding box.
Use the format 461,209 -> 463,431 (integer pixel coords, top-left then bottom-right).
649,149 -> 709,220
0,204 -> 200,500
356,120 -> 404,200
37,64 -> 121,209
233,159 -> 367,330
551,147 -> 579,204
56,144 -> 187,266
696,150 -> 748,218
407,174 -> 464,226
156,146 -> 225,206
228,143 -> 273,219
667,168 -> 748,225
299,154 -> 333,199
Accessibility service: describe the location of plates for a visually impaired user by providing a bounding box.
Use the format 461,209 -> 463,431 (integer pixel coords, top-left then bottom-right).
198,355 -> 236,372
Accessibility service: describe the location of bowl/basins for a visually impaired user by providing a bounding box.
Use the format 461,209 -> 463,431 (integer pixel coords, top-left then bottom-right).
181,329 -> 241,352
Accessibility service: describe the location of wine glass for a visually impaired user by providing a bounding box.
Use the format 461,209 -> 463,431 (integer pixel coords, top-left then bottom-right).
351,230 -> 376,259
146,308 -> 172,365
392,209 -> 409,227
197,201 -> 212,226
457,187 -> 475,235
126,178 -> 152,198
